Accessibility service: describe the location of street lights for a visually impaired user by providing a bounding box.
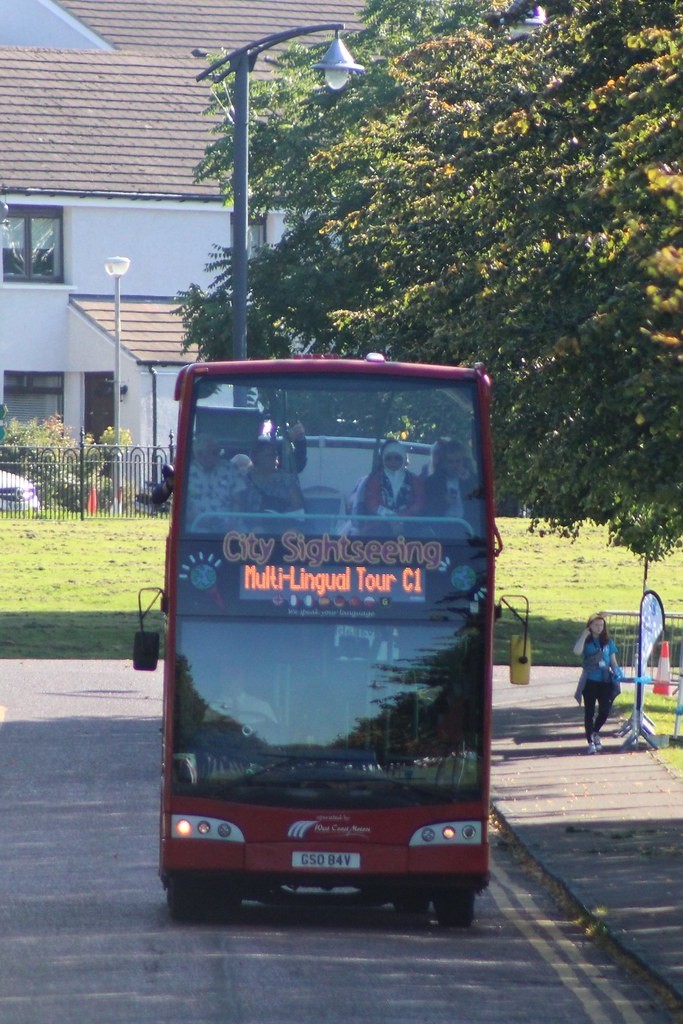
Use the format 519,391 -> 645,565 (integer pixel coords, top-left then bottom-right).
197,23 -> 364,404
104,256 -> 132,502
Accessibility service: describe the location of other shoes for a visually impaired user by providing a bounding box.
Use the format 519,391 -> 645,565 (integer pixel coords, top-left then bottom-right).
588,745 -> 595,753
594,735 -> 602,751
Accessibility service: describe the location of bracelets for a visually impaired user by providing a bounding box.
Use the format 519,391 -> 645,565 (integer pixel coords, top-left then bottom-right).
165,479 -> 173,492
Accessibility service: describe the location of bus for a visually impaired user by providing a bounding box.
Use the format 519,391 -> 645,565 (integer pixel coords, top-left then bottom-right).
135,354 -> 533,926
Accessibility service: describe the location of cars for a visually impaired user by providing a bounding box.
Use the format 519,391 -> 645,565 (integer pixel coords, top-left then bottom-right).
0,470 -> 41,512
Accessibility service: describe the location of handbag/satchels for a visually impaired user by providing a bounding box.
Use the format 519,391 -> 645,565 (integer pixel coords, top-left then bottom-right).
260,495 -> 293,525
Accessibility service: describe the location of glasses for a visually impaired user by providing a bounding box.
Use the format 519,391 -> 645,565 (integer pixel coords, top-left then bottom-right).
212,446 -> 223,457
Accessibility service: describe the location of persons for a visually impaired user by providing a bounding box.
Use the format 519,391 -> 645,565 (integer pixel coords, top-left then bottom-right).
150,417 -> 307,533
200,664 -> 279,729
572,612 -> 624,755
361,437 -> 484,530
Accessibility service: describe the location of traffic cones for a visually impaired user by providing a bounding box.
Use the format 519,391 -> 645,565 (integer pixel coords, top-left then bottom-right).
86,488 -> 98,514
652,640 -> 672,698
